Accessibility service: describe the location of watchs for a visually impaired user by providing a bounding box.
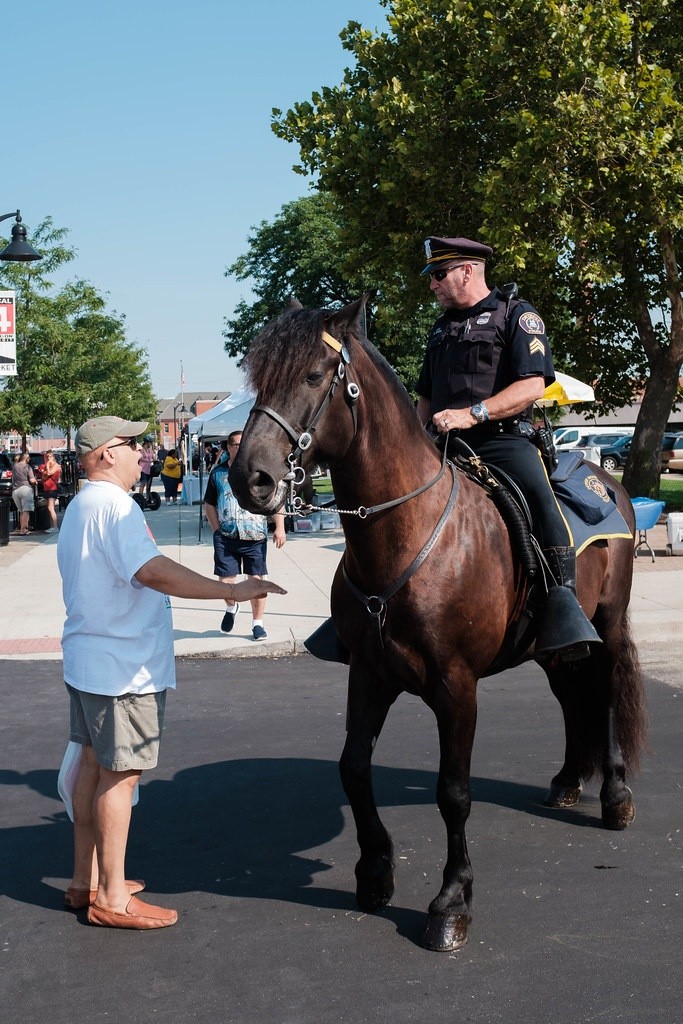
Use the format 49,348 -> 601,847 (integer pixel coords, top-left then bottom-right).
470,404 -> 484,424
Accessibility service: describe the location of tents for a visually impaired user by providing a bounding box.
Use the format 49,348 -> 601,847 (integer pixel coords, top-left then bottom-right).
188,386 -> 259,543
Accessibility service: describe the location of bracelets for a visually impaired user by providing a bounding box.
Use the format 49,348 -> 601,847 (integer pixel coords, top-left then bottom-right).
225,583 -> 235,607
478,402 -> 489,421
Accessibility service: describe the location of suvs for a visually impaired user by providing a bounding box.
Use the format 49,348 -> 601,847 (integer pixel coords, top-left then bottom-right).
0,453 -> 15,496
18,448 -> 83,483
574,433 -> 628,450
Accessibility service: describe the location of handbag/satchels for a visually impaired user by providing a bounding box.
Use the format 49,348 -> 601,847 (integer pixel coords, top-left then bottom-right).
57,484 -> 63,494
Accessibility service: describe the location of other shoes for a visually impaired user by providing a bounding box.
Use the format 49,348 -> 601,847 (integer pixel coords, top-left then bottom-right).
173,500 -> 182,505
165,501 -> 172,506
89,893 -> 178,929
45,527 -> 60,533
64,878 -> 146,910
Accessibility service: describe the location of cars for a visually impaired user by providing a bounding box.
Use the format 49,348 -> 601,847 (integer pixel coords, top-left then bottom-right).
661,433 -> 683,473
598,436 -> 635,472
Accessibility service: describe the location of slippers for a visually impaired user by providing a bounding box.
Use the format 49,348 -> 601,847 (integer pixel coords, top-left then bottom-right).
18,530 -> 31,536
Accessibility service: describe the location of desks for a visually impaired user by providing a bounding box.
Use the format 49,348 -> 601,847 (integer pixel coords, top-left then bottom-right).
179,475 -> 210,506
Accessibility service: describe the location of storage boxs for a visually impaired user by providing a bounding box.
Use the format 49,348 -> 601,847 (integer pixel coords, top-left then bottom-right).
293,494 -> 341,532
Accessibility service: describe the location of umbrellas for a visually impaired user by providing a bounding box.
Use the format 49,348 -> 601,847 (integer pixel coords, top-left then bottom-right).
533,371 -> 595,408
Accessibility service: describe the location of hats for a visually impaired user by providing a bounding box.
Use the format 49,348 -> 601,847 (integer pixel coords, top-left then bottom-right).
74,416 -> 149,458
418,236 -> 496,277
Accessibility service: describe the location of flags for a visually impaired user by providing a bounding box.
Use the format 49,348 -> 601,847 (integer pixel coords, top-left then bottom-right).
180,365 -> 184,384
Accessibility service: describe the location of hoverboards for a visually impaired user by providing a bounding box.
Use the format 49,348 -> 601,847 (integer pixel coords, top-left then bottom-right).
131,460 -> 162,511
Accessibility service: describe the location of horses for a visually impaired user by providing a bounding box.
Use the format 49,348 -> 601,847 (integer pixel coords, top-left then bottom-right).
227,290 -> 650,952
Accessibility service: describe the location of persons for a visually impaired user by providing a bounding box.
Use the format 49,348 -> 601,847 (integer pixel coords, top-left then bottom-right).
155,444 -> 167,462
56,416 -> 288,929
40,450 -> 60,533
415,236 -> 575,592
204,431 -> 286,641
161,449 -> 182,506
192,440 -> 230,470
12,453 -> 38,535
139,435 -> 164,504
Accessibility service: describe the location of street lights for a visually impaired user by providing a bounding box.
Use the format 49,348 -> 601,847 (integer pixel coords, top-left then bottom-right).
172,401 -> 188,449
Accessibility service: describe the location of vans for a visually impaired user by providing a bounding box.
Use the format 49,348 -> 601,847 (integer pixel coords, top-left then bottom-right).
552,426 -> 635,451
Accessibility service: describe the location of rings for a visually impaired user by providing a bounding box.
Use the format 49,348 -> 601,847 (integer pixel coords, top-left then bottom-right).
443,420 -> 448,425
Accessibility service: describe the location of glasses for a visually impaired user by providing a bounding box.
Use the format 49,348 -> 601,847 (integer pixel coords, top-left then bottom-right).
428,262 -> 479,282
230,442 -> 240,449
100,436 -> 138,461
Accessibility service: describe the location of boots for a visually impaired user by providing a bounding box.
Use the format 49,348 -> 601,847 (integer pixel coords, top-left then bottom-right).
539,547 -> 591,663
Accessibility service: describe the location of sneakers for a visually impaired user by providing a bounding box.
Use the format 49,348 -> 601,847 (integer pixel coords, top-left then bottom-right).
252,625 -> 266,641
220,602 -> 240,633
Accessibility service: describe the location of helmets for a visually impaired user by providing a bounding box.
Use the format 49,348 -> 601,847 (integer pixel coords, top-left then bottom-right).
143,436 -> 153,442
